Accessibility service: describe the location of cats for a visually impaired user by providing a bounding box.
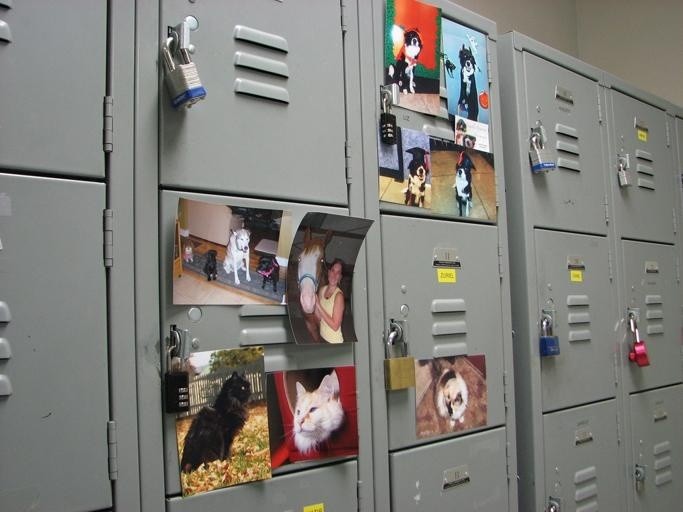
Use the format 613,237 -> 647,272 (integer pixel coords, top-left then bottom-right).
281,373 -> 344,455
181,370 -> 253,475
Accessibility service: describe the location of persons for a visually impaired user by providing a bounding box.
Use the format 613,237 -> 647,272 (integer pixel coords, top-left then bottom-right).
313,256 -> 346,344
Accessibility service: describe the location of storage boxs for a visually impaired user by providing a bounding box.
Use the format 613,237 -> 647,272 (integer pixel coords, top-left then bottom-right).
524,47 -> 609,236
1,174 -> 107,510
627,383 -> 682,511
371,1 -> 499,225
156,3 -> 352,201
378,210 -> 508,451
389,425 -> 509,511
604,87 -> 677,244
618,236 -> 682,395
156,184 -> 368,496
532,226 -> 618,412
540,399 -> 627,512
166,456 -> 358,511
1,1 -> 114,175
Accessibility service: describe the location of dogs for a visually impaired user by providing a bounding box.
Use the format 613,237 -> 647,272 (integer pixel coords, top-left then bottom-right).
222,227 -> 252,287
402,158 -> 429,208
452,161 -> 473,217
256,254 -> 280,293
455,43 -> 480,121
386,30 -> 423,96
202,249 -> 218,282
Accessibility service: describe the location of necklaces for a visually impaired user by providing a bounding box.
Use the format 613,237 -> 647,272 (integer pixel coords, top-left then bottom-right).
326,285 -> 333,299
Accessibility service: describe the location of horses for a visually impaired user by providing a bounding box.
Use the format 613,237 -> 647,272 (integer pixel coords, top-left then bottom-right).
295,224 -> 334,342
